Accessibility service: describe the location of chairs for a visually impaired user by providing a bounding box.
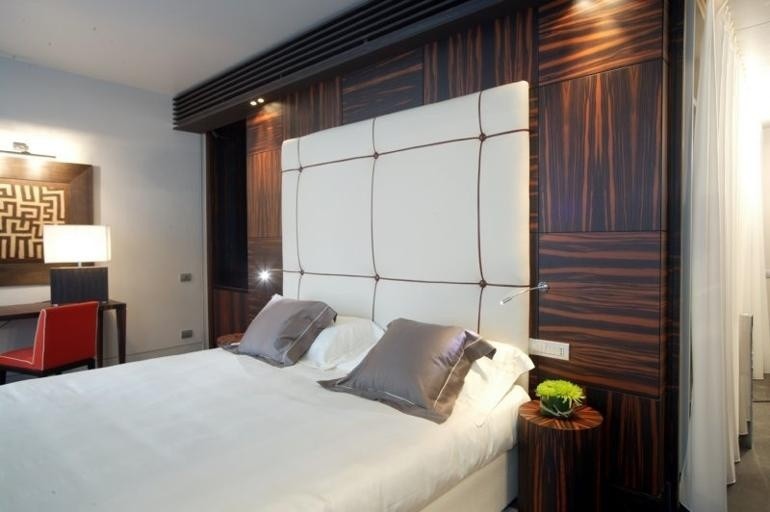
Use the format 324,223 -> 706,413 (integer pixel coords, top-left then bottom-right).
1,301 -> 99,385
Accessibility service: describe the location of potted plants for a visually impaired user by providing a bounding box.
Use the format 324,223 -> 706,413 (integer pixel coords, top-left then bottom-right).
535,378 -> 586,420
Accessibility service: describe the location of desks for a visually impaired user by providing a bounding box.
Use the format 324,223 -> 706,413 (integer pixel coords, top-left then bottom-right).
0,300 -> 127,384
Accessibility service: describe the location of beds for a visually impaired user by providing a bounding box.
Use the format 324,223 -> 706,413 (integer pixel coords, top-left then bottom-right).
0,292 -> 533,512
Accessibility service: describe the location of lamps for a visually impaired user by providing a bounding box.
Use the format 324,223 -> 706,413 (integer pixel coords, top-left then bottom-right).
43,224 -> 111,307
